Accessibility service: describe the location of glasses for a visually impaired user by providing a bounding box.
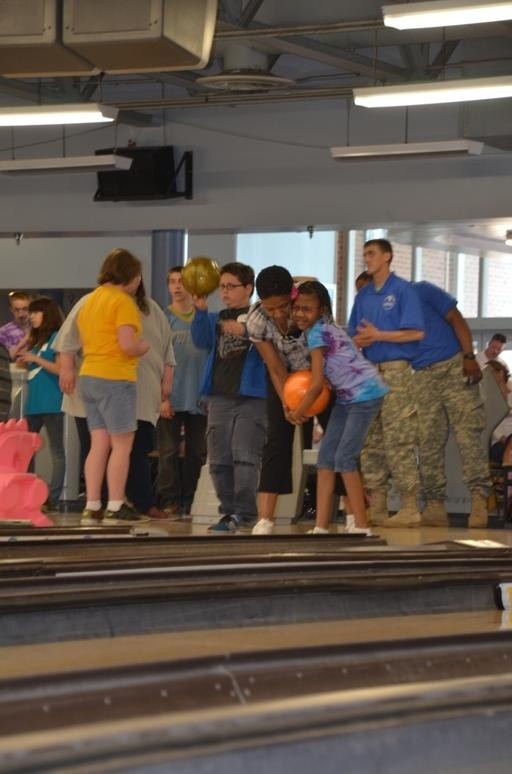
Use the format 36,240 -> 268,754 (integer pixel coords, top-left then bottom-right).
220,282 -> 242,290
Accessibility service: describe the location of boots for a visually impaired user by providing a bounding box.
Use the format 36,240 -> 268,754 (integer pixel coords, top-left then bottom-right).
469,493 -> 488,528
366,490 -> 389,527
384,490 -> 421,527
419,499 -> 449,527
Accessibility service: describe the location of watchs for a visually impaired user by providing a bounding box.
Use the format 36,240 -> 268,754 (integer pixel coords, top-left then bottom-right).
463,353 -> 476,360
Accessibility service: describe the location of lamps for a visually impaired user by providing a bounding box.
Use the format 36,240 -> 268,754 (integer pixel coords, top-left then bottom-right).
0,121 -> 135,174
381,0 -> 512,30
0,71 -> 120,128
329,96 -> 469,158
351,30 -> 512,109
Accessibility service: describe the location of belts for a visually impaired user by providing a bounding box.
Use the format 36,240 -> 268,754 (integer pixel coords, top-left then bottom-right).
427,360 -> 448,370
375,359 -> 408,372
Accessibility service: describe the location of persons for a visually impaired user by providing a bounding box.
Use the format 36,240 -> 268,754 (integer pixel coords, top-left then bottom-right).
356,271 -> 493,530
0,291 -> 32,420
347,237 -> 423,527
16,297 -> 67,513
477,333 -> 510,371
50,249 -> 390,538
483,359 -> 511,514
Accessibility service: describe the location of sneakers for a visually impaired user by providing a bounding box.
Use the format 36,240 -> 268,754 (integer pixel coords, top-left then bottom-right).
207,514 -> 274,535
79,503 -> 192,525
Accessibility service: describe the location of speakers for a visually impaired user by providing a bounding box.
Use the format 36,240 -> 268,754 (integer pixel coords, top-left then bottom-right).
93,145 -> 177,201
